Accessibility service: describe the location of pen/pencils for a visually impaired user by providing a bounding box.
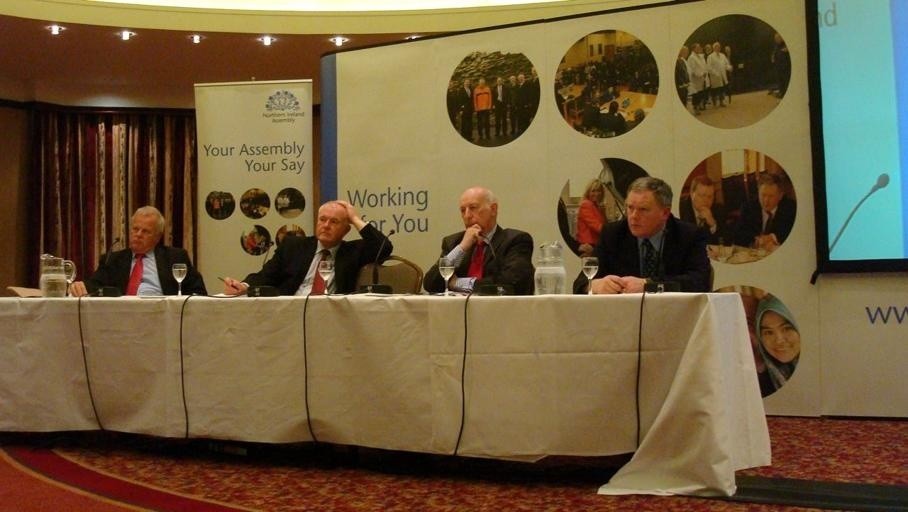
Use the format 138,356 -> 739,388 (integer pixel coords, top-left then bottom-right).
218,276 -> 239,290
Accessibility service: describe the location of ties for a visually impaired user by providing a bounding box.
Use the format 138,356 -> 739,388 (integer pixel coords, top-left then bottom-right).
126,254 -> 145,295
467,240 -> 484,281
766,211 -> 772,229
312,250 -> 330,295
642,240 -> 657,277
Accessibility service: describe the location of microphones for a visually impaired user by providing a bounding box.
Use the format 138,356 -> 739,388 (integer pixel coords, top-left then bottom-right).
89,236 -> 122,297
247,240 -> 278,296
359,228 -> 396,293
478,230 -> 515,296
829,171 -> 890,257
643,227 -> 682,292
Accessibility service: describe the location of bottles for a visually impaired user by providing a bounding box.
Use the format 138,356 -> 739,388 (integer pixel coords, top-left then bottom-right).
716,236 -> 728,263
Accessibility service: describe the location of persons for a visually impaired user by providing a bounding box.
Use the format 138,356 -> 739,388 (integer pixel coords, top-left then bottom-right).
755,294 -> 808,398
675,29 -> 790,120
679,173 -> 732,246
568,178 -> 712,303
424,186 -> 535,300
219,200 -> 394,300
65,205 -> 211,300
731,172 -> 797,251
553,40 -> 660,139
577,180 -> 616,260
447,66 -> 545,143
205,186 -> 305,256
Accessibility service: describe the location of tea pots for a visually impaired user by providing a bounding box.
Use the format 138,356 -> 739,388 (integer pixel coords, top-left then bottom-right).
534,241 -> 567,295
39,254 -> 76,298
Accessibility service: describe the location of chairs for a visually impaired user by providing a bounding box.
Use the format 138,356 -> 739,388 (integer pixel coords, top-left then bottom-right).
356,256 -> 423,294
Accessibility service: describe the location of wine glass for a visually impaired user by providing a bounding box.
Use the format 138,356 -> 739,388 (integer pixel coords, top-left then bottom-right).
582,257 -> 599,295
439,259 -> 456,296
172,263 -> 188,298
318,261 -> 336,296
